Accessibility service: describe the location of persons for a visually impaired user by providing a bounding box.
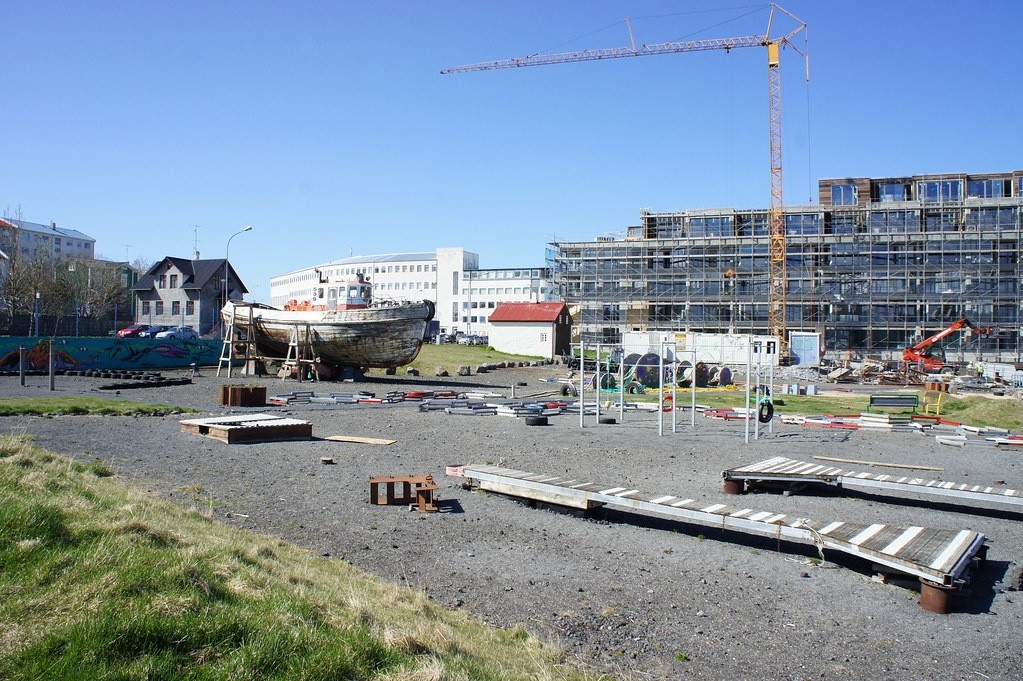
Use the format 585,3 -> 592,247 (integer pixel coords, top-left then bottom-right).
976,362 -> 984,378
966,361 -> 973,374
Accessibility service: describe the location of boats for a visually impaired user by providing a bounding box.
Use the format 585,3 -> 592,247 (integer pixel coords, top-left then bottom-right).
219,267 -> 435,370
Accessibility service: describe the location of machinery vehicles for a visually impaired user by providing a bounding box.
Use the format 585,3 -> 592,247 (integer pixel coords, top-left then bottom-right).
901,317 -> 992,376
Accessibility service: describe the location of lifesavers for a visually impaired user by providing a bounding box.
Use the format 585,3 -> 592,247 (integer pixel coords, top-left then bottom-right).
662,395 -> 673,412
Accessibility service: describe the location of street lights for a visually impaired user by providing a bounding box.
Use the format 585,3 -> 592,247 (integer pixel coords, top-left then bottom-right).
225,225 -> 253,303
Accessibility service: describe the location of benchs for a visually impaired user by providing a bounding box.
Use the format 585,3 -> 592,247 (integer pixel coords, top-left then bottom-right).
867,394 -> 918,415
918,391 -> 942,415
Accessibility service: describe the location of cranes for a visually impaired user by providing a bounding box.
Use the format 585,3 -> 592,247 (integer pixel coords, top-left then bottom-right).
439,2 -> 814,365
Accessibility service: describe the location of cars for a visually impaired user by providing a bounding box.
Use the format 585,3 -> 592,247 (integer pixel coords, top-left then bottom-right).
155,326 -> 199,341
138,324 -> 178,340
423,326 -> 489,348
115,324 -> 152,339
